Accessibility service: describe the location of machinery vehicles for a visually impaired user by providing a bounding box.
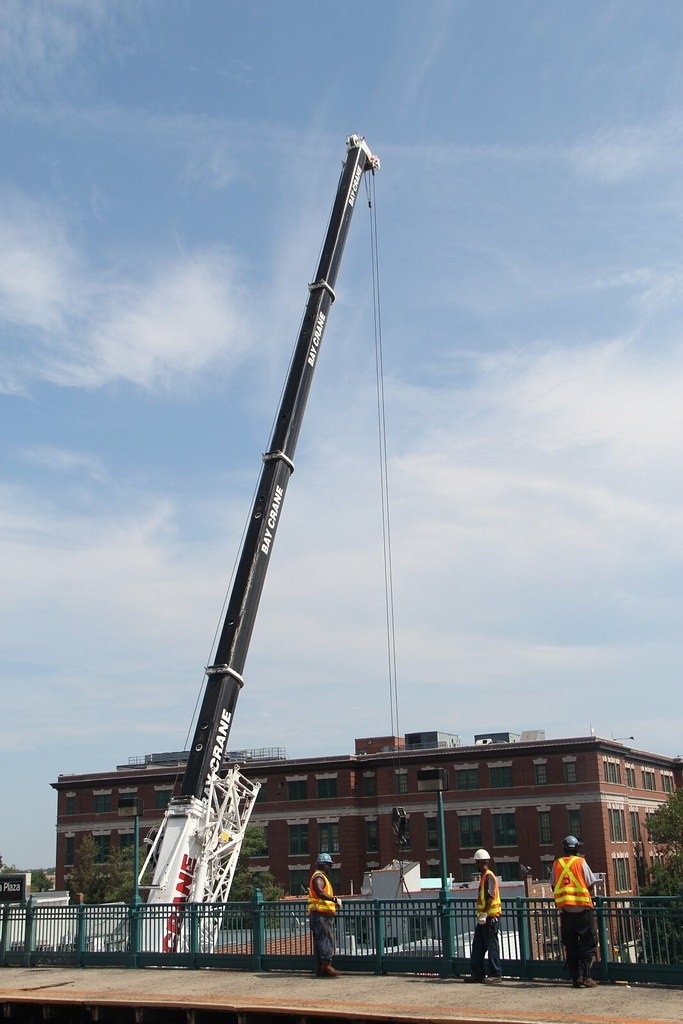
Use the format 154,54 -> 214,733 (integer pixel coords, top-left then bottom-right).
92,132 -> 403,959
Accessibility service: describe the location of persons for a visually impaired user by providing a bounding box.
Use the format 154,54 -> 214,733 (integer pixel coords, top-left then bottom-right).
307,852 -> 342,978
464,849 -> 503,984
550,835 -> 599,989
289,913 -> 299,934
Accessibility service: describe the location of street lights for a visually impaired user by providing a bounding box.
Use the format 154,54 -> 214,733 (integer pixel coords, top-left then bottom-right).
118,796 -> 146,967
416,767 -> 460,978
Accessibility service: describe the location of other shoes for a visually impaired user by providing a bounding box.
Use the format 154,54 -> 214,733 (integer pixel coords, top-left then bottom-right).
316,962 -> 341,977
483,977 -> 502,984
464,975 -> 484,983
572,976 -> 597,989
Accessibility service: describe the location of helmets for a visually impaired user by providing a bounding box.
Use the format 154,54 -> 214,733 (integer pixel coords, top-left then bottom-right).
563,835 -> 579,847
316,853 -> 334,866
474,849 -> 490,860
